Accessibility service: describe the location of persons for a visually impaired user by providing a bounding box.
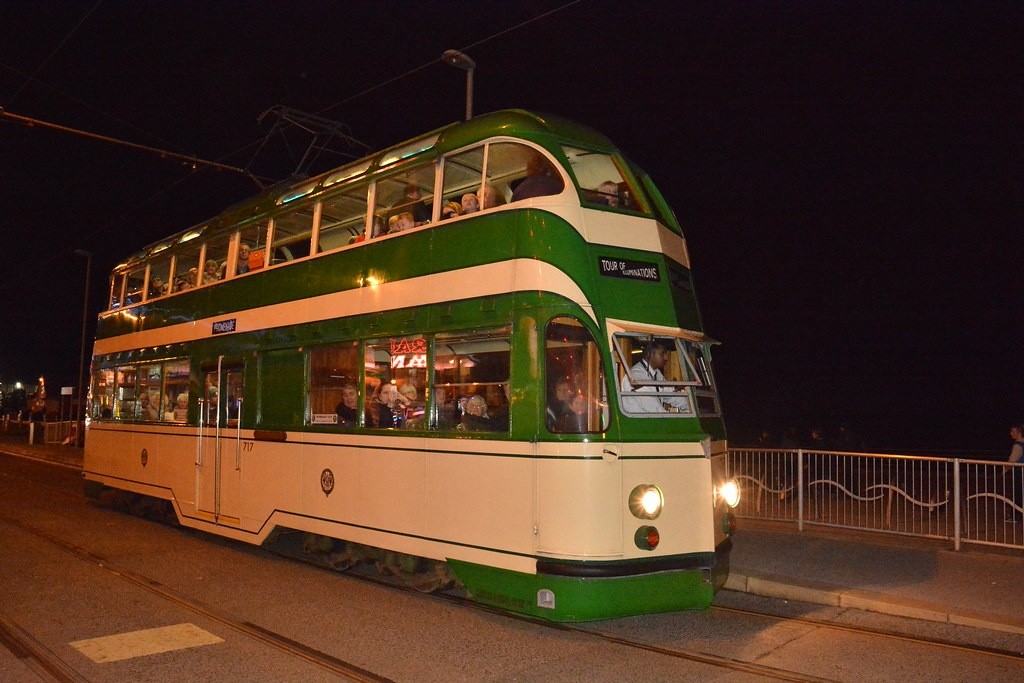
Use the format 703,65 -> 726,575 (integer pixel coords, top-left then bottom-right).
207,386 -> 243,419
622,341 -> 689,413
335,382 -> 425,429
125,243 -> 250,306
435,380 -> 507,432
759,423 -> 864,500
441,185 -> 507,220
546,380 -> 588,432
510,153 -> 612,205
600,181 -> 626,208
102,401 -> 134,419
138,393 -> 188,421
1002,426 -> 1024,523
354,185 -> 431,243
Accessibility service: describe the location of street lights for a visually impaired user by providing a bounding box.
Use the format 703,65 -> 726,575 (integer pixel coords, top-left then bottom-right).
440,49 -> 476,122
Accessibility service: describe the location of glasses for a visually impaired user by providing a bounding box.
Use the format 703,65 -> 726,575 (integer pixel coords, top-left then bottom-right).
152,279 -> 160,283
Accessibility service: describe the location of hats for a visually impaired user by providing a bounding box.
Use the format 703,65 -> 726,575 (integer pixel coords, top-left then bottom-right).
208,385 -> 218,398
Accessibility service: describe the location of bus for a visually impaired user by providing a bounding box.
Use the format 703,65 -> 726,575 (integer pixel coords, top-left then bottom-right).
80,107 -> 742,627
